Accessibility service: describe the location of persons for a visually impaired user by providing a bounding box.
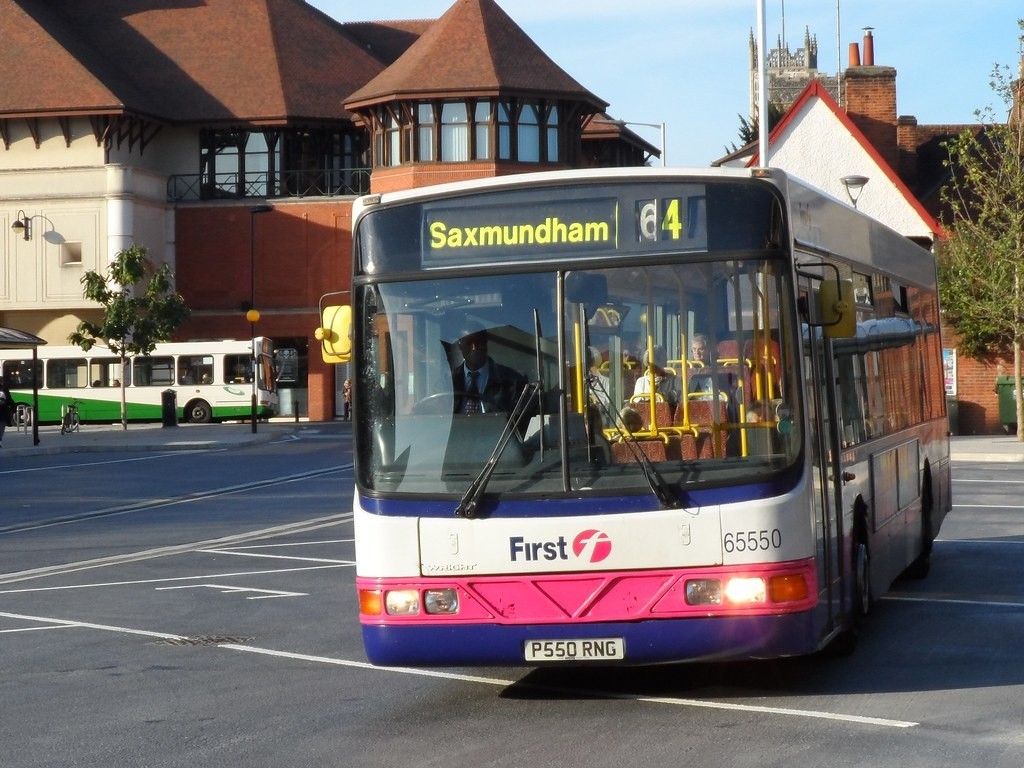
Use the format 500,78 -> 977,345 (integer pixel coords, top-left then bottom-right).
688,330 -> 717,400
747,401 -> 772,423
404,320 -> 539,440
113,377 -> 120,387
586,344 -> 611,409
0,376 -> 17,448
340,378 -> 352,423
633,345 -> 682,414
614,406 -> 643,442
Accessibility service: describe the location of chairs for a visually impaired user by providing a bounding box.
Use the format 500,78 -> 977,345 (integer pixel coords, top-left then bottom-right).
609,334 -> 783,461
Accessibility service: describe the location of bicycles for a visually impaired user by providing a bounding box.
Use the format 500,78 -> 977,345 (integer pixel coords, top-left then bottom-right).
61,401 -> 85,435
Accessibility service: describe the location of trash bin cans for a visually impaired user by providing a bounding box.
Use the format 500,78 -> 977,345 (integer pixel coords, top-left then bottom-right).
945,395 -> 959,435
162,389 -> 178,426
997,376 -> 1024,424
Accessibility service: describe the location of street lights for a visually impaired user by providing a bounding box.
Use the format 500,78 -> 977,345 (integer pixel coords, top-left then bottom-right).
594,119 -> 665,167
249,206 -> 273,309
247,310 -> 259,433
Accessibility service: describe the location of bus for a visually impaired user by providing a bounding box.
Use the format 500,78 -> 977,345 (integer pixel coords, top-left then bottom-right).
0,337 -> 280,423
315,166 -> 952,667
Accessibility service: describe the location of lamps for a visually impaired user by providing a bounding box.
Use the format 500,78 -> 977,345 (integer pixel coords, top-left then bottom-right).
838,175 -> 869,210
10,210 -> 29,241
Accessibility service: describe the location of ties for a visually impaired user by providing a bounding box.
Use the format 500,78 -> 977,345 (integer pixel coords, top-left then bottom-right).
463,371 -> 481,414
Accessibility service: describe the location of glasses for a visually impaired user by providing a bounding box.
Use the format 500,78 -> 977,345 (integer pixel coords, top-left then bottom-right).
690,347 -> 709,353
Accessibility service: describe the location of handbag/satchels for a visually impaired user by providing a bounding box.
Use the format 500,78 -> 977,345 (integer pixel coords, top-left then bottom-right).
348,402 -> 352,412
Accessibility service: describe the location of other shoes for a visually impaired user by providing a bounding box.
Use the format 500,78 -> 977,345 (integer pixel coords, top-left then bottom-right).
0,442 -> 3,448
